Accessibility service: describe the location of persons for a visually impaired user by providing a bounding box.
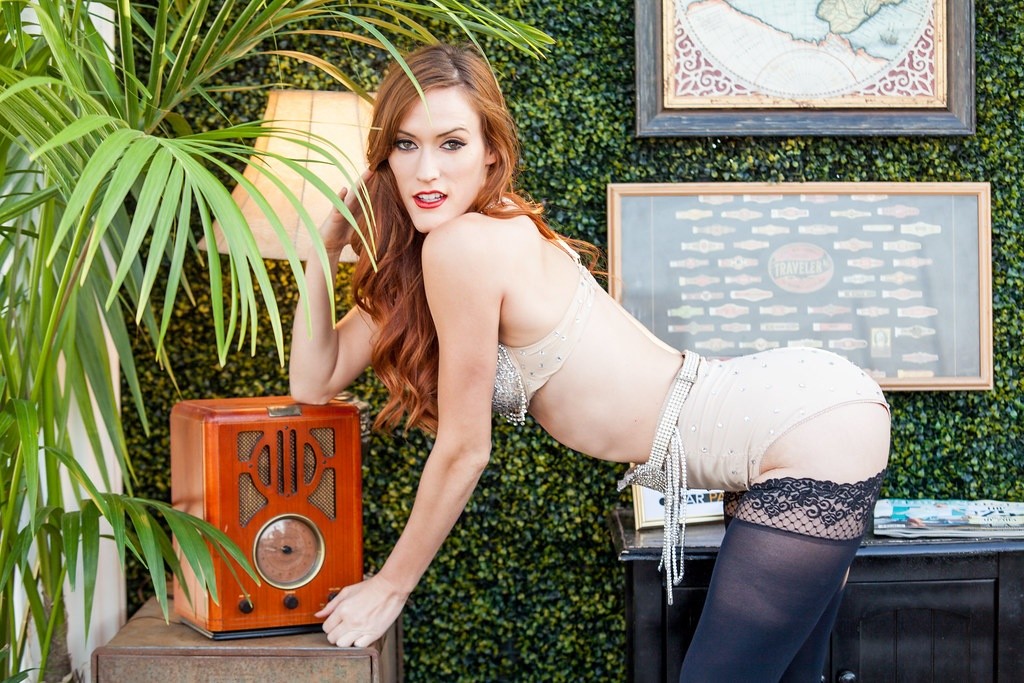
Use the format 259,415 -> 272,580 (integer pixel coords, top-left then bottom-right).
289,43 -> 889,683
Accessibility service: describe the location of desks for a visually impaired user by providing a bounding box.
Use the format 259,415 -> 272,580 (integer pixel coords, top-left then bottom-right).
90,582 -> 404,683
608,508 -> 1024,683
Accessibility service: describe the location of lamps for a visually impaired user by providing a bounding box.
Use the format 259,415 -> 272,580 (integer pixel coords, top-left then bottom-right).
195,85 -> 379,261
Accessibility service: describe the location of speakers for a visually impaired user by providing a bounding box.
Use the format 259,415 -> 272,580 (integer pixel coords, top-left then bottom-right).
171,395 -> 364,631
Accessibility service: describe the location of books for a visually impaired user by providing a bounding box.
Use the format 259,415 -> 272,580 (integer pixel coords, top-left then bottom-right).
871,497 -> 1024,542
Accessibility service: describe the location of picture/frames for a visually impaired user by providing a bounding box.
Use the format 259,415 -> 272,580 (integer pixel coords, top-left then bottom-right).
631,462 -> 724,531
632,0 -> 976,138
605,182 -> 996,394
